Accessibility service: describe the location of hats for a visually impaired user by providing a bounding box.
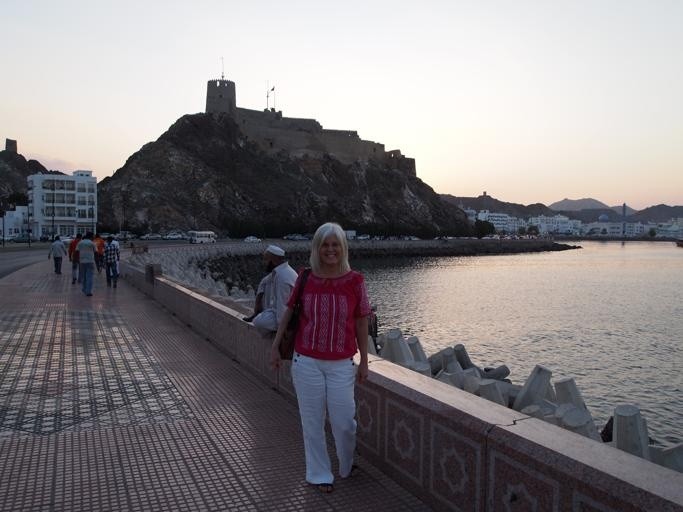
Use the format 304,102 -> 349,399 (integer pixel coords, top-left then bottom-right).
265,245 -> 285,257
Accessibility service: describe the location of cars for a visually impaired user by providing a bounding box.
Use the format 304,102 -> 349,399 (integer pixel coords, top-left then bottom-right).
47,233 -> 77,245
244,236 -> 262,243
0,233 -> 37,243
98,231 -> 188,241
357,233 -> 549,241
280,232 -> 312,241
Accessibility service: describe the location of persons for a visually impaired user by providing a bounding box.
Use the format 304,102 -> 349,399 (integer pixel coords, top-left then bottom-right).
46,236 -> 67,274
241,244 -> 298,337
67,232 -> 122,299
265,221 -> 372,493
367,307 -> 379,352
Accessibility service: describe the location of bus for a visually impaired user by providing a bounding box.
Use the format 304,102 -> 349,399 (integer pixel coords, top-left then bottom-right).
187,231 -> 218,244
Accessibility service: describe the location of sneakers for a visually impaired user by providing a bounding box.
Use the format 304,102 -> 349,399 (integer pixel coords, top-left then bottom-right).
87,293 -> 92,296
72,278 -> 77,284
106,281 -> 118,288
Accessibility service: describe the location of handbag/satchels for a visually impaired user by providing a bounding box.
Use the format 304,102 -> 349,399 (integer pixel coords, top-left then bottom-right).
278,268 -> 309,361
